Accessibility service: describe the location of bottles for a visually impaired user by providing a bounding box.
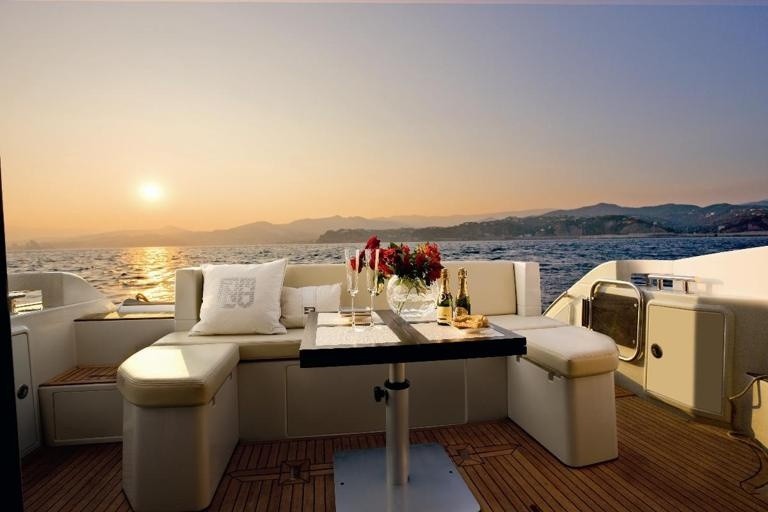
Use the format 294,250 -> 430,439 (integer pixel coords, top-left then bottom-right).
436,266 -> 470,326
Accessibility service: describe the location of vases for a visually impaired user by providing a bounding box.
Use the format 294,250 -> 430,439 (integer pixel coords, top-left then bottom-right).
386,273 -> 442,318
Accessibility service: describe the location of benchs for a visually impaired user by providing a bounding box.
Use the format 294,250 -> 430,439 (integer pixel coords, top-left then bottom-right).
36,366 -> 125,445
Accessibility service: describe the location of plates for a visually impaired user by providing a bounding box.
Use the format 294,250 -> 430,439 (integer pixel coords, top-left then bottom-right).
447,321 -> 491,334
337,305 -> 371,321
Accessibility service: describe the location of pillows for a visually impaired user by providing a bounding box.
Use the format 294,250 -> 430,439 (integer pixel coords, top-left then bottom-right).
188,255 -> 342,337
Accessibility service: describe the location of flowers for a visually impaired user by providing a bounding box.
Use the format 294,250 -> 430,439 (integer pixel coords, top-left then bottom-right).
350,234 -> 445,316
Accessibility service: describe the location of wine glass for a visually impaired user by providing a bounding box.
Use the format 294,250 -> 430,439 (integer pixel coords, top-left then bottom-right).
343,248 -> 380,333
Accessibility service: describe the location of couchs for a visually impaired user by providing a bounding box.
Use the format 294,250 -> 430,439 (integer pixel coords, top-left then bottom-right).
115,260 -> 620,512
